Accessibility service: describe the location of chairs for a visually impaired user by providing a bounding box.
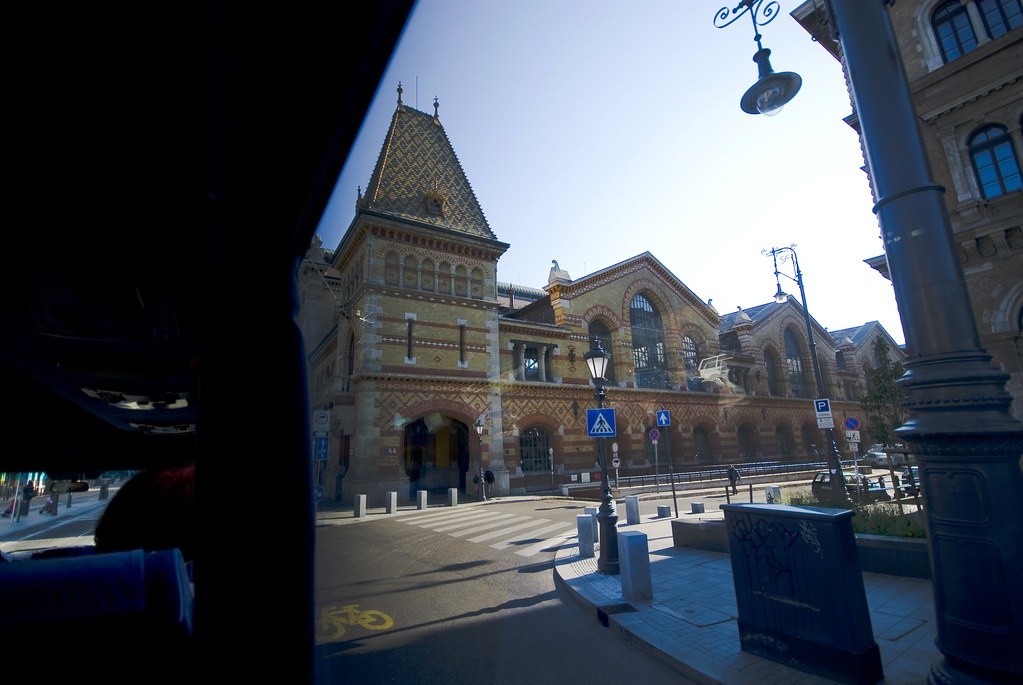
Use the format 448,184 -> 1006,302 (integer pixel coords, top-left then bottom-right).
0,547 -> 194,685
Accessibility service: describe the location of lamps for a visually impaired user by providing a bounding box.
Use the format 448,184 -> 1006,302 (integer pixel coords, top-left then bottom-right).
571,401 -> 579,415
760,408 -> 768,420
756,371 -> 761,381
723,407 -> 730,420
567,345 -> 576,365
712,0 -> 803,117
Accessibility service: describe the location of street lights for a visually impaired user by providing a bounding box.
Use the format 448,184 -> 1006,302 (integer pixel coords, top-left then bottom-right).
584,336 -> 620,575
770,247 -> 854,508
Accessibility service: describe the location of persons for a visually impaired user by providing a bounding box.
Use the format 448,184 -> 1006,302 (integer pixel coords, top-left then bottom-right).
728,464 -> 741,495
50,481 -> 56,500
2,481 -> 33,517
474,466 -> 495,500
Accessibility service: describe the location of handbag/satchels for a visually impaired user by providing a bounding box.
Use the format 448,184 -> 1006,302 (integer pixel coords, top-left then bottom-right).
473,473 -> 479,484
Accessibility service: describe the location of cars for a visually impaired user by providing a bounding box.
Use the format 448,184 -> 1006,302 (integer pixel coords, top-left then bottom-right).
812,471 -> 891,503
902,467 -> 921,496
861,452 -> 898,469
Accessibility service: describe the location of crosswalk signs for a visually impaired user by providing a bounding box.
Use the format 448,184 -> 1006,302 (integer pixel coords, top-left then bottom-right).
586,408 -> 617,437
